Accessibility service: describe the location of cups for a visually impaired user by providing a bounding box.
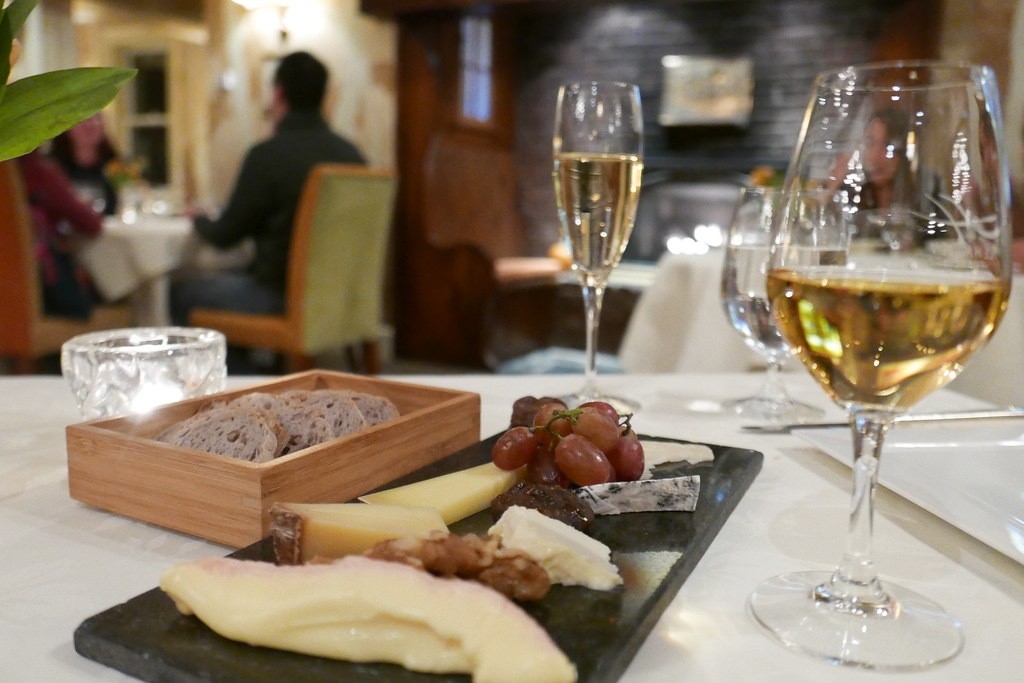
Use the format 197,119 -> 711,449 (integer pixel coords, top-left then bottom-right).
61,325 -> 228,425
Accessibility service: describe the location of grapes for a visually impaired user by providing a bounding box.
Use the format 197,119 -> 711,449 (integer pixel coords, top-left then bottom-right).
493,397 -> 646,485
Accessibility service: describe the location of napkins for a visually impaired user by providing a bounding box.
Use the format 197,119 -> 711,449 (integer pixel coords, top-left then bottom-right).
612,245 -> 781,404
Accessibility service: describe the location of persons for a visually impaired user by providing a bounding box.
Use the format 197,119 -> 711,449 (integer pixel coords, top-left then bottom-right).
962,114 -> 1024,273
18,154 -> 105,320
167,51 -> 367,375
819,106 -> 946,249
46,113 -> 125,216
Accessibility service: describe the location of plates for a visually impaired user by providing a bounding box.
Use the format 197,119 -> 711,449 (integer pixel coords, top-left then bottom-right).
791,418 -> 1023,563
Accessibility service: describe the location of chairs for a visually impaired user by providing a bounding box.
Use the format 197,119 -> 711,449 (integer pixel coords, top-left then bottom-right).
1,164 -> 141,376
187,162 -> 395,370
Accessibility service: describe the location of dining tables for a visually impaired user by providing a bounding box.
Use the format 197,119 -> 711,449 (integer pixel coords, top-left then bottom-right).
81,218 -> 202,330
1,368 -> 1024,683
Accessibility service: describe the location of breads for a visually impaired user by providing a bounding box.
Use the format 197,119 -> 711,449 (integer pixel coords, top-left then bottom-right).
152,387 -> 400,463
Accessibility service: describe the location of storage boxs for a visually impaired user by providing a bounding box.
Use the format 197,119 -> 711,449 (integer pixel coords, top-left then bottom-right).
65,368 -> 480,547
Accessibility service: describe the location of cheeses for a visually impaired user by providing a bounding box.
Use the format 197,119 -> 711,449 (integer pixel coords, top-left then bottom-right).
269,452 -> 527,563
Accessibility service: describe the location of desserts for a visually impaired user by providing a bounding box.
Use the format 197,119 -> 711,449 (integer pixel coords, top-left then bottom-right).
487,506 -> 624,594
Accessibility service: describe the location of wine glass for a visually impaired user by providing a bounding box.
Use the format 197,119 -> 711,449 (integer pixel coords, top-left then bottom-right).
722,187 -> 847,424
752,60 -> 1014,669
552,82 -> 645,416
